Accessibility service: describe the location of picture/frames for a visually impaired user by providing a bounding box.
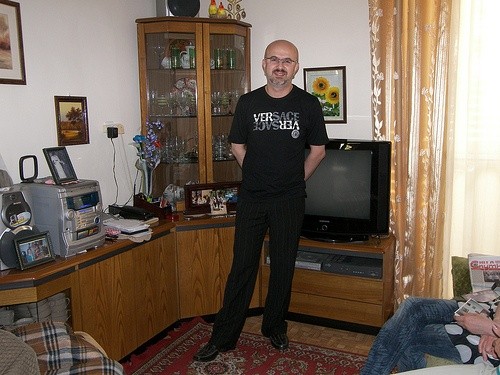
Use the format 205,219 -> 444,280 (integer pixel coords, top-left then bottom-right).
184,181 -> 241,215
43,147 -> 78,185
54,96 -> 90,146
303,66 -> 347,124
0,0 -> 27,85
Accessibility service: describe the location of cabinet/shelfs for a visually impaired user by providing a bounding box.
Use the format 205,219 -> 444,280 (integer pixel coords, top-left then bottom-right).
136,17 -> 252,212
0,216 -> 396,363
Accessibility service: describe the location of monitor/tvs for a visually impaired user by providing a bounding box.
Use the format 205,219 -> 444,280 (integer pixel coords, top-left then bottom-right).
299,138 -> 391,243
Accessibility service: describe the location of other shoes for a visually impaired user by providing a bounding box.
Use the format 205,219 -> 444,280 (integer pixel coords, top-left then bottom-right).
193,344 -> 230,362
269,332 -> 289,349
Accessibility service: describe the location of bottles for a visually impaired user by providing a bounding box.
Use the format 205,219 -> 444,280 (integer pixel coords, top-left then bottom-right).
209,0 -> 226,15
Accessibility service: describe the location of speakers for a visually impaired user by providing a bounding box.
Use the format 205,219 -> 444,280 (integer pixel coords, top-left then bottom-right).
0,185 -> 35,271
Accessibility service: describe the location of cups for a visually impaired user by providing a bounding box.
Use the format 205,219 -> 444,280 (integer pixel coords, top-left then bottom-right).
161,53 -> 216,70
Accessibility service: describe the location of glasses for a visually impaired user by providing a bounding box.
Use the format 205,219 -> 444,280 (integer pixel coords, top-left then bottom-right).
266,56 -> 295,64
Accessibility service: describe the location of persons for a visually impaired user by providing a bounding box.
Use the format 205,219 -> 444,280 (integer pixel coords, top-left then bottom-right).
359,275 -> 500,375
193,40 -> 331,360
21,239 -> 49,264
51,151 -> 72,180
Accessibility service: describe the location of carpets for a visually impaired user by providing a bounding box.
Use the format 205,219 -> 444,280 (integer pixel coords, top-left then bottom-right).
123,316 -> 397,375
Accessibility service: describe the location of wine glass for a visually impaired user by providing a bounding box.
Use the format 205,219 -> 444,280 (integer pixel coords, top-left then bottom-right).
152,88 -> 242,161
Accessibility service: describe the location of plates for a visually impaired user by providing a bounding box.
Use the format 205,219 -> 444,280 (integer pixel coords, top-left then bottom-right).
210,15 -> 228,19
167,0 -> 201,17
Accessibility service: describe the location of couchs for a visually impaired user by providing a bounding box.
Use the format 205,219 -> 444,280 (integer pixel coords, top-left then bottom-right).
451,256 -> 473,298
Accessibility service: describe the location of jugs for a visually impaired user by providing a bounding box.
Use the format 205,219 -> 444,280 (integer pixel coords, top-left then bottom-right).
180,45 -> 195,57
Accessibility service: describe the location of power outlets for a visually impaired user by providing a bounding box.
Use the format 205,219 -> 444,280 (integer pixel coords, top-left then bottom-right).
104,121 -> 125,134
